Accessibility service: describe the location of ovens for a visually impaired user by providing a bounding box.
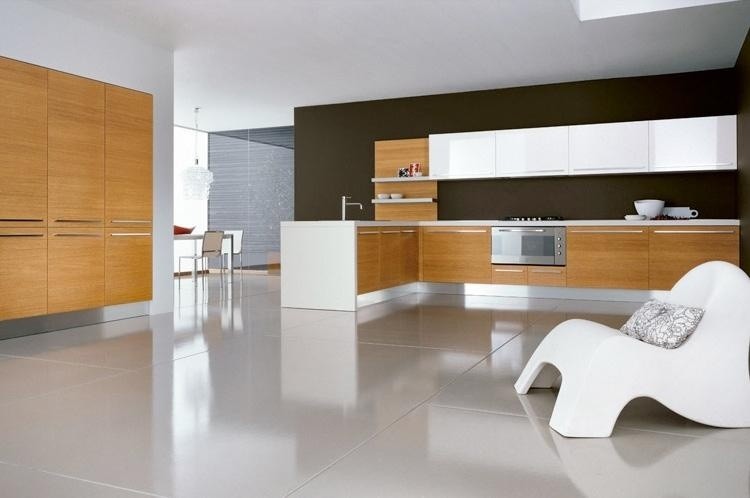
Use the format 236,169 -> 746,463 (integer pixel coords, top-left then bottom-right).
490,226 -> 566,267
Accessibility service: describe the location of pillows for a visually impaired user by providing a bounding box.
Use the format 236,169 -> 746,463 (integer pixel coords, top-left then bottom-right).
618,297 -> 707,350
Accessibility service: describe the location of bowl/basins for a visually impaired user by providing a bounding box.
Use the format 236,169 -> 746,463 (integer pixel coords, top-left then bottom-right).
634,199 -> 665,219
378,193 -> 390,199
391,194 -> 404,199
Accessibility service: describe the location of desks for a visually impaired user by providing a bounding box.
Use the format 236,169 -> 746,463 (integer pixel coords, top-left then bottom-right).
175,233 -> 236,285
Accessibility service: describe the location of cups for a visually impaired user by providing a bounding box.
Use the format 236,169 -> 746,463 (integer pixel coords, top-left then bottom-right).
664,206 -> 699,219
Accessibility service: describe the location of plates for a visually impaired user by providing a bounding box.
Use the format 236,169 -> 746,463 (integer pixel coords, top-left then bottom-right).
624,215 -> 646,221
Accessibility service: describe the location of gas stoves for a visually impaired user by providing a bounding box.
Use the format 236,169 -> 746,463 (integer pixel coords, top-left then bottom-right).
507,216 -> 562,221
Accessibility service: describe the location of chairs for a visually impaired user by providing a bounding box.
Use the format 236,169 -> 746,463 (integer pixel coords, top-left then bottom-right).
513,254 -> 750,443
179,228 -> 246,289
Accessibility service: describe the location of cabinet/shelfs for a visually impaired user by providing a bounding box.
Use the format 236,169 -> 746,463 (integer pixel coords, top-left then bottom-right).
569,120 -> 648,175
567,228 -> 648,293
280,225 -> 421,310
496,125 -> 569,178
428,130 -> 496,180
491,264 -> 568,287
649,227 -> 739,292
648,114 -> 747,173
0,54 -> 156,321
368,175 -> 434,205
419,228 -> 492,286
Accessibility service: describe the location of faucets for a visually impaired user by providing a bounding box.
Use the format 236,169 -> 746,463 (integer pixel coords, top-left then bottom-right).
341,195 -> 363,221
